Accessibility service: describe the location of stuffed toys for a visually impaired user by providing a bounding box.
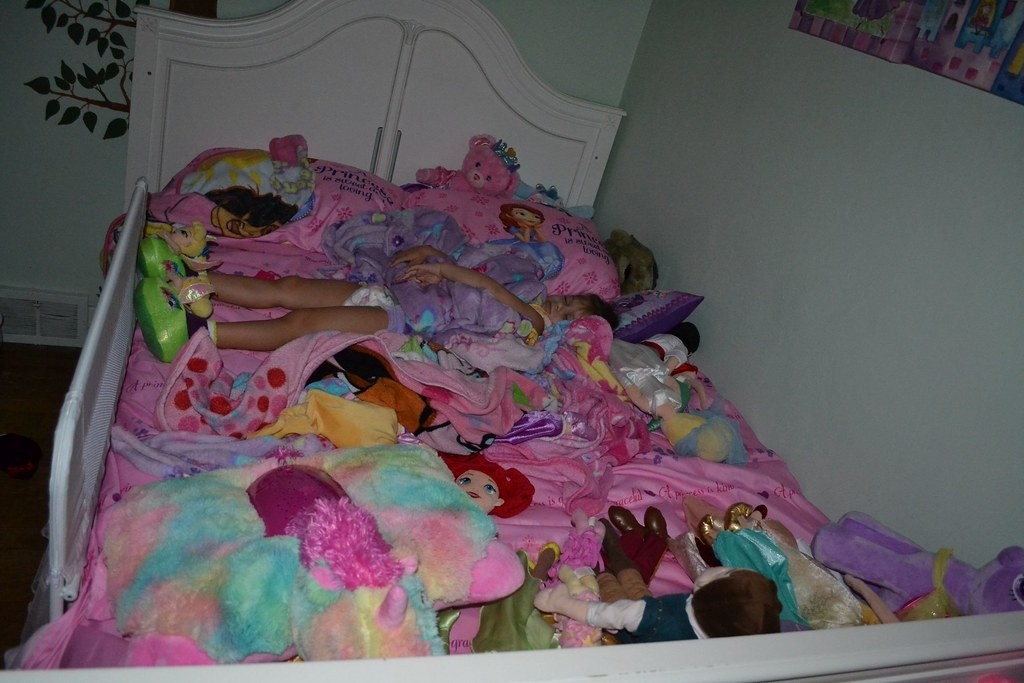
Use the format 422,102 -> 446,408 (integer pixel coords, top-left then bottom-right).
414,130 -> 519,195
101,321 -> 1022,663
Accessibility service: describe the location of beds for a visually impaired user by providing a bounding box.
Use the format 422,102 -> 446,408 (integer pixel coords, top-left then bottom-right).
0,0 -> 1024,683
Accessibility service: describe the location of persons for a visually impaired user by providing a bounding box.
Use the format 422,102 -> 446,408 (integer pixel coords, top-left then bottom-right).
133,221 -> 615,375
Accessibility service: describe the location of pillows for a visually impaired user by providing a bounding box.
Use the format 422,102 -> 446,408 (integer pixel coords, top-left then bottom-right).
607,289 -> 704,342
402,188 -> 625,306
93,440 -> 528,649
144,146 -> 410,261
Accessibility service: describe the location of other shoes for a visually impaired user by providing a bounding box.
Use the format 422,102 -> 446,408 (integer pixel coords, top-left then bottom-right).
139,236 -> 197,291
136,277 -> 190,363
